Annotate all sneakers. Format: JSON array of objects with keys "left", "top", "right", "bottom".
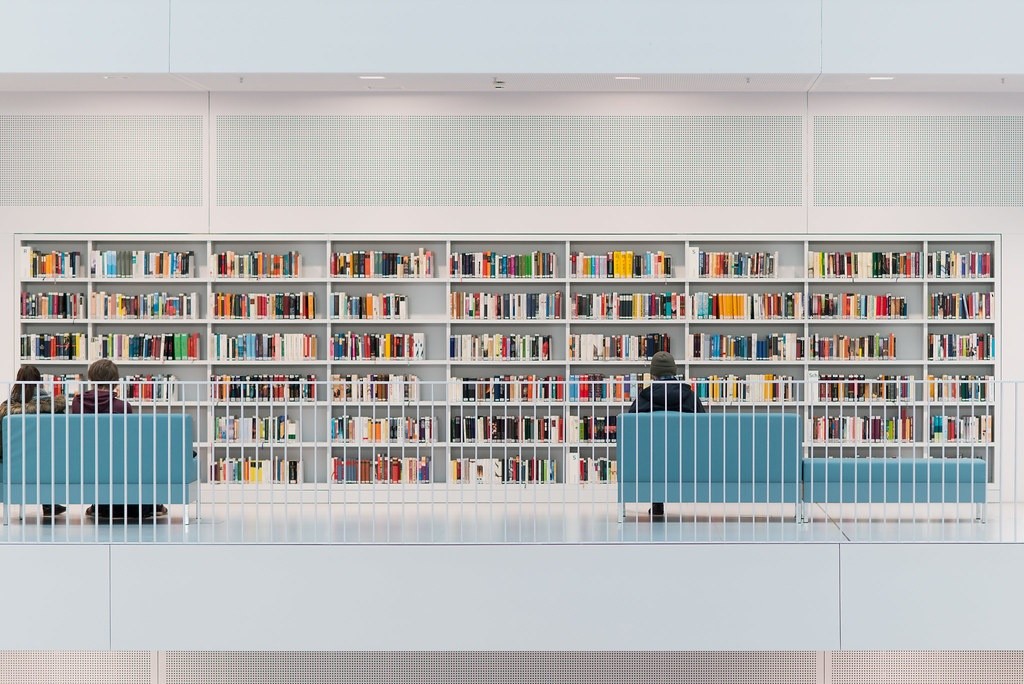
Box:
[
  {"left": 85, "top": 505, "right": 102, "bottom": 516},
  {"left": 648, "top": 503, "right": 664, "bottom": 515},
  {"left": 43, "top": 505, "right": 67, "bottom": 516},
  {"left": 154, "top": 506, "right": 168, "bottom": 515}
]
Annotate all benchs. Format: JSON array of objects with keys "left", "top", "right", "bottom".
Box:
[
  {"left": 1, "top": 413, "right": 201, "bottom": 525},
  {"left": 616, "top": 413, "right": 803, "bottom": 523},
  {"left": 802, "top": 458, "right": 988, "bottom": 524}
]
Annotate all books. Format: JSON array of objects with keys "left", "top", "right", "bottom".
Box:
[{"left": 19, "top": 248, "right": 997, "bottom": 483}]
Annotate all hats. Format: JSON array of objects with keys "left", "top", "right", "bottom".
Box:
[{"left": 650, "top": 351, "right": 677, "bottom": 376}]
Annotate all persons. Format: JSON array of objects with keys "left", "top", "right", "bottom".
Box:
[
  {"left": 0, "top": 365, "right": 67, "bottom": 517},
  {"left": 71, "top": 358, "right": 167, "bottom": 519},
  {"left": 628, "top": 350, "right": 706, "bottom": 516}
]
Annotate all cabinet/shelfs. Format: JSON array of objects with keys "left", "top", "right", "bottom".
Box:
[
  {"left": 329, "top": 233, "right": 450, "bottom": 506},
  {"left": 13, "top": 233, "right": 91, "bottom": 413},
  {"left": 806, "top": 235, "right": 927, "bottom": 457},
  {"left": 927, "top": 234, "right": 1002, "bottom": 490},
  {"left": 211, "top": 233, "right": 331, "bottom": 505},
  {"left": 566, "top": 236, "right": 686, "bottom": 504},
  {"left": 448, "top": 236, "right": 566, "bottom": 506},
  {"left": 685, "top": 236, "right": 808, "bottom": 458},
  {"left": 90, "top": 233, "right": 212, "bottom": 504}
]
